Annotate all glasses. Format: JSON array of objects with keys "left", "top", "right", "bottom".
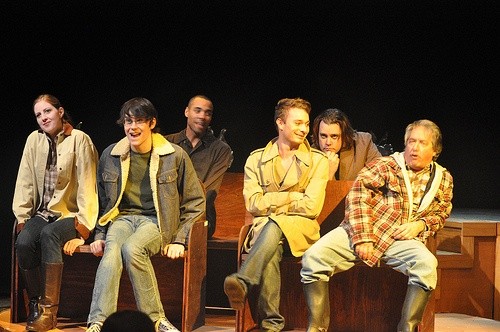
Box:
[{"left": 122, "top": 117, "right": 151, "bottom": 126}]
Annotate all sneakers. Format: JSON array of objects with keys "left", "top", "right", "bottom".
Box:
[
  {"left": 154, "top": 318, "right": 181, "bottom": 332},
  {"left": 85, "top": 321, "right": 102, "bottom": 332}
]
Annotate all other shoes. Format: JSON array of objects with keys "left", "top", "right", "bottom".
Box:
[
  {"left": 247, "top": 325, "right": 274, "bottom": 332},
  {"left": 224, "top": 275, "right": 249, "bottom": 310}
]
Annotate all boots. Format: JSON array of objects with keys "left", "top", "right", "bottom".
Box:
[
  {"left": 25, "top": 263, "right": 63, "bottom": 332},
  {"left": 18, "top": 264, "right": 42, "bottom": 324},
  {"left": 302, "top": 281, "right": 330, "bottom": 332},
  {"left": 397, "top": 284, "right": 432, "bottom": 332}
]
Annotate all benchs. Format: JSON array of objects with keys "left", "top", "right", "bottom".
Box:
[
  {"left": 235, "top": 181, "right": 435, "bottom": 332},
  {"left": 10, "top": 178, "right": 207, "bottom": 332}
]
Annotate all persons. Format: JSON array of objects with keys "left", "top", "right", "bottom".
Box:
[
  {"left": 166, "top": 95, "right": 233, "bottom": 239},
  {"left": 301, "top": 120, "right": 453, "bottom": 332},
  {"left": 311, "top": 111, "right": 383, "bottom": 181},
  {"left": 86, "top": 99, "right": 206, "bottom": 332},
  {"left": 12, "top": 94, "right": 99, "bottom": 332},
  {"left": 224, "top": 99, "right": 329, "bottom": 332},
  {"left": 102, "top": 310, "right": 156, "bottom": 332}
]
